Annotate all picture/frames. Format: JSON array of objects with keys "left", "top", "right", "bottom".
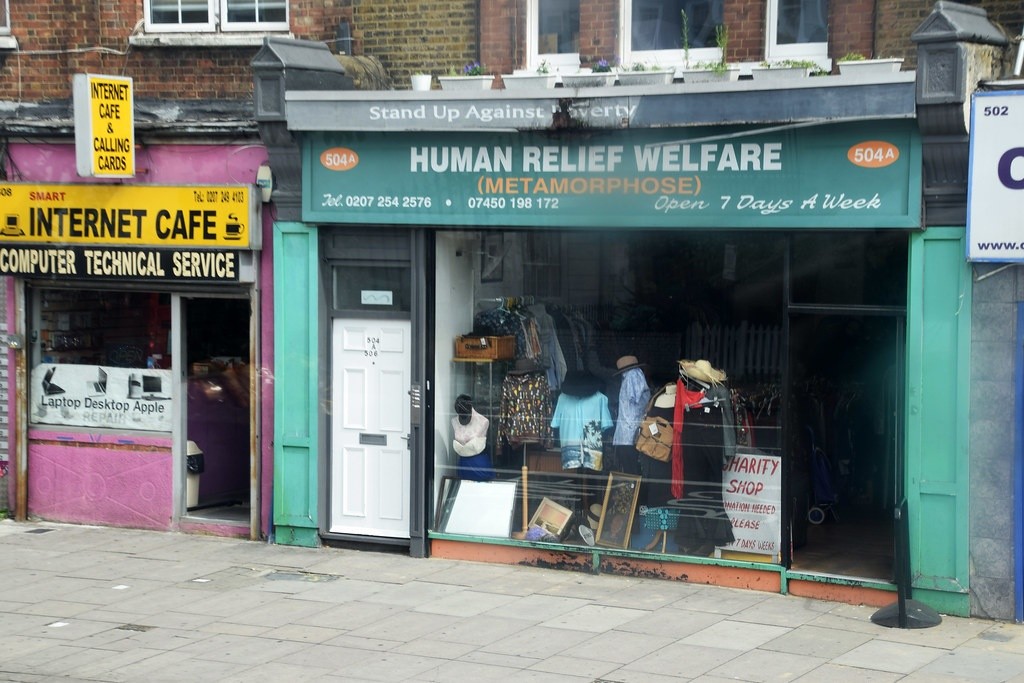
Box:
[
  {"left": 595, "top": 470, "right": 642, "bottom": 549},
  {"left": 481, "top": 232, "right": 504, "bottom": 283},
  {"left": 528, "top": 497, "right": 573, "bottom": 535}
]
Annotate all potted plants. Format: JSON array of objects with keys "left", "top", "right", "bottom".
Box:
[
  {"left": 835, "top": 50, "right": 905, "bottom": 75},
  {"left": 681, "top": 9, "right": 740, "bottom": 84},
  {"left": 500, "top": 58, "right": 560, "bottom": 90},
  {"left": 751, "top": 57, "right": 828, "bottom": 79},
  {"left": 410, "top": 71, "right": 432, "bottom": 91}
]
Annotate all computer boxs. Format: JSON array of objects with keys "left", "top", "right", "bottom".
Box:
[{"left": 128, "top": 373, "right": 142, "bottom": 399}]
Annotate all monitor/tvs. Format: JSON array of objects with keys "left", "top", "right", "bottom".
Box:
[{"left": 143, "top": 375, "right": 162, "bottom": 397}]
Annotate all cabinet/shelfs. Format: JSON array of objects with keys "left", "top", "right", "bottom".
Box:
[{"left": 40, "top": 309, "right": 108, "bottom": 356}]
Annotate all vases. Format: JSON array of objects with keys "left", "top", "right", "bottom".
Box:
[
  {"left": 438, "top": 75, "right": 496, "bottom": 91},
  {"left": 618, "top": 71, "right": 675, "bottom": 86},
  {"left": 560, "top": 74, "right": 616, "bottom": 88}
]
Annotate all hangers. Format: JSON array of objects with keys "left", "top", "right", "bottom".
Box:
[
  {"left": 492, "top": 296, "right": 583, "bottom": 320},
  {"left": 731, "top": 382, "right": 780, "bottom": 420},
  {"left": 685, "top": 389, "right": 727, "bottom": 412}
]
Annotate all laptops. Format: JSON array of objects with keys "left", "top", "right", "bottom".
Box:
[
  {"left": 86, "top": 367, "right": 108, "bottom": 395},
  {"left": 42, "top": 367, "right": 65, "bottom": 395}
]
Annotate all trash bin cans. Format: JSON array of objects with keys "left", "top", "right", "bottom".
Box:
[{"left": 187, "top": 440, "right": 203, "bottom": 508}]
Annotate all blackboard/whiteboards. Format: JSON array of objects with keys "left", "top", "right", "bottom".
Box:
[{"left": 714, "top": 453, "right": 782, "bottom": 554}]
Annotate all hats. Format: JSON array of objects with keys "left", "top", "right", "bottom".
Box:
[
  {"left": 655, "top": 385, "right": 677, "bottom": 408},
  {"left": 561, "top": 369, "right": 601, "bottom": 397},
  {"left": 588, "top": 504, "right": 601, "bottom": 530},
  {"left": 507, "top": 359, "right": 545, "bottom": 377},
  {"left": 454, "top": 394, "right": 473, "bottom": 425},
  {"left": 612, "top": 356, "right": 646, "bottom": 378},
  {"left": 680, "top": 359, "right": 727, "bottom": 382}
]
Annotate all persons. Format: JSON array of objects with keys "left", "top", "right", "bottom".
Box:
[
  {"left": 638, "top": 384, "right": 703, "bottom": 551},
  {"left": 452, "top": 394, "right": 489, "bottom": 456},
  {"left": 612, "top": 356, "right": 651, "bottom": 472},
  {"left": 551, "top": 370, "right": 613, "bottom": 472},
  {"left": 496, "top": 359, "right": 555, "bottom": 456}
]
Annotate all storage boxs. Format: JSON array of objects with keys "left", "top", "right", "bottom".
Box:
[{"left": 455, "top": 335, "right": 517, "bottom": 360}]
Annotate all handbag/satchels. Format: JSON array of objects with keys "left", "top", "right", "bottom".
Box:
[{"left": 634, "top": 416, "right": 674, "bottom": 462}]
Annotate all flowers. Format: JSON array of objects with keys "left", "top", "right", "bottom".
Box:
[
  {"left": 592, "top": 58, "right": 612, "bottom": 73},
  {"left": 445, "top": 61, "right": 486, "bottom": 76},
  {"left": 619, "top": 54, "right": 676, "bottom": 71}
]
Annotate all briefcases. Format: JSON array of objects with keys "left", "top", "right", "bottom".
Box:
[{"left": 455, "top": 335, "right": 516, "bottom": 359}]
